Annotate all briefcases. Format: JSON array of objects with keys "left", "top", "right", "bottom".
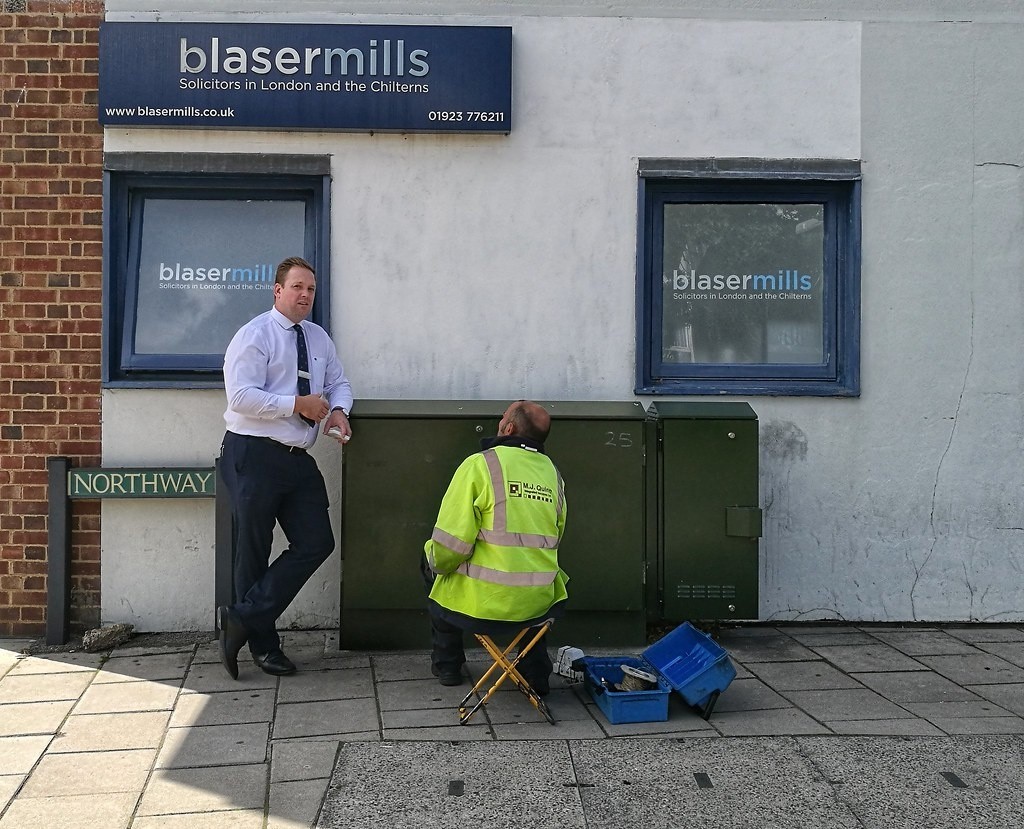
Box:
[{"left": 580, "top": 620, "right": 738, "bottom": 727}]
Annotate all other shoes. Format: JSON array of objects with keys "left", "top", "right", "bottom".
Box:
[
  {"left": 524, "top": 679, "right": 550, "bottom": 696},
  {"left": 431, "top": 664, "right": 462, "bottom": 686}
]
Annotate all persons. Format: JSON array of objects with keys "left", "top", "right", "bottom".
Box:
[
  {"left": 216, "top": 258, "right": 354, "bottom": 679},
  {"left": 420, "top": 399, "right": 571, "bottom": 698}
]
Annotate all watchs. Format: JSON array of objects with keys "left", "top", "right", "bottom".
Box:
[{"left": 330, "top": 406, "right": 350, "bottom": 419}]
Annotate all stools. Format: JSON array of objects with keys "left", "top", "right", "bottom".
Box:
[{"left": 455, "top": 618, "right": 561, "bottom": 731}]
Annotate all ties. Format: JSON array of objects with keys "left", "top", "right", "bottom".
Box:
[{"left": 292, "top": 324, "right": 315, "bottom": 428}]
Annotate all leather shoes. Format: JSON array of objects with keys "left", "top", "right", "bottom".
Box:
[
  {"left": 252, "top": 649, "right": 296, "bottom": 675},
  {"left": 217, "top": 605, "right": 247, "bottom": 679}
]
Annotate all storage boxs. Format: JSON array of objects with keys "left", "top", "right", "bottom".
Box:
[{"left": 582, "top": 618, "right": 741, "bottom": 725}]
